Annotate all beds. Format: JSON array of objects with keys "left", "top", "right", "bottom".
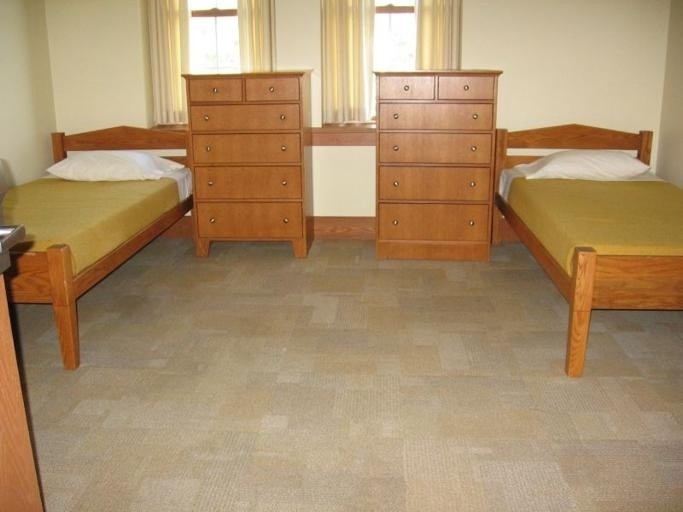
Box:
[
  {"left": 0, "top": 122, "right": 193, "bottom": 373},
  {"left": 488, "top": 123, "right": 682, "bottom": 384}
]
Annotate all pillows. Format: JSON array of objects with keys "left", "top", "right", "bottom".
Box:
[
  {"left": 45, "top": 149, "right": 185, "bottom": 184},
  {"left": 512, "top": 143, "right": 655, "bottom": 184}
]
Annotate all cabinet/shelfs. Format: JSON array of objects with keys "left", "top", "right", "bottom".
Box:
[
  {"left": 175, "top": 64, "right": 318, "bottom": 259},
  {"left": 368, "top": 66, "right": 505, "bottom": 266}
]
